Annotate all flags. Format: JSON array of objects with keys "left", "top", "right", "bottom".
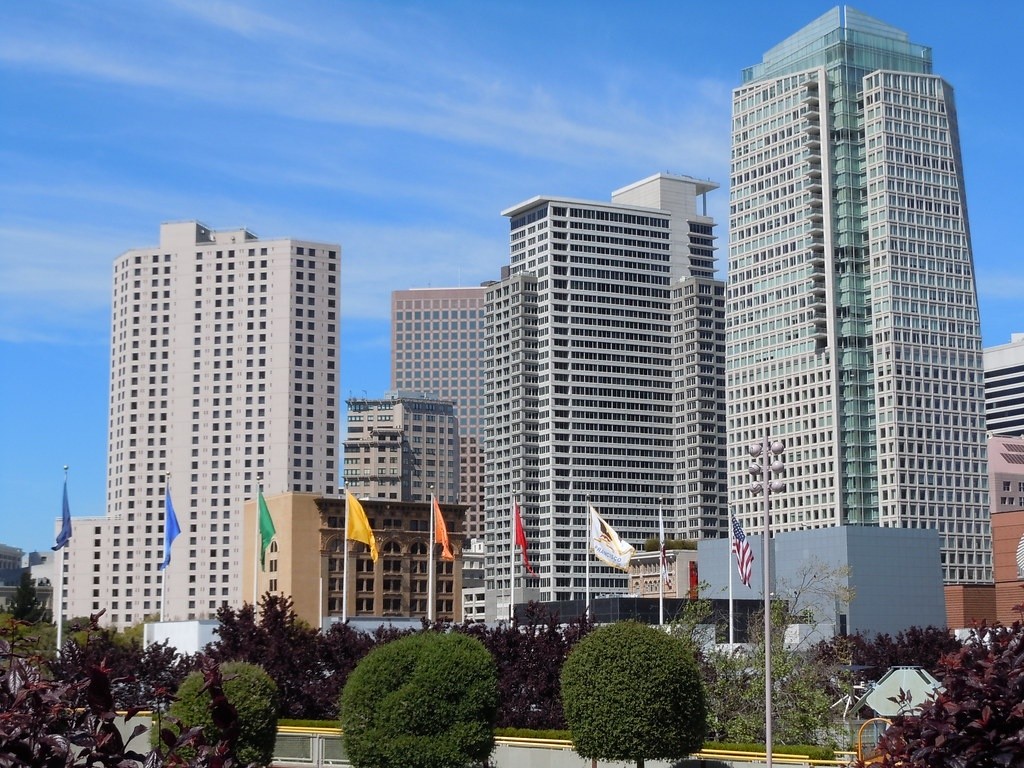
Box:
[
  {"left": 515, "top": 504, "right": 528, "bottom": 565},
  {"left": 345, "top": 491, "right": 378, "bottom": 564},
  {"left": 660, "top": 511, "right": 672, "bottom": 589},
  {"left": 589, "top": 506, "right": 635, "bottom": 572},
  {"left": 258, "top": 492, "right": 275, "bottom": 572},
  {"left": 161, "top": 486, "right": 181, "bottom": 570},
  {"left": 731, "top": 510, "right": 754, "bottom": 589},
  {"left": 51, "top": 483, "right": 72, "bottom": 551},
  {"left": 434, "top": 497, "right": 455, "bottom": 560}
]
